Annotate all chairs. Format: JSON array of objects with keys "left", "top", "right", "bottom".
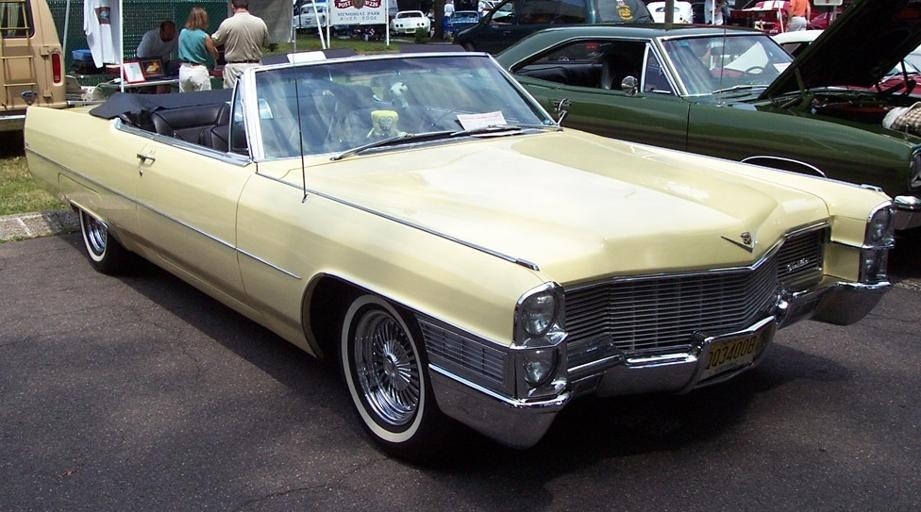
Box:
[
  {"left": 519, "top": 44, "right": 736, "bottom": 99},
  {"left": 144, "top": 82, "right": 414, "bottom": 159}
]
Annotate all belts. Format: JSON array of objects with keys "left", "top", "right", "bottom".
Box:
[
  {"left": 227, "top": 59, "right": 259, "bottom": 63},
  {"left": 179, "top": 59, "right": 203, "bottom": 65}
]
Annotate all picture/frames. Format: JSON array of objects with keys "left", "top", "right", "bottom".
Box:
[{"left": 122, "top": 57, "right": 168, "bottom": 83}]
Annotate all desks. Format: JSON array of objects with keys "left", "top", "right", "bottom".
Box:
[{"left": 101, "top": 76, "right": 185, "bottom": 102}]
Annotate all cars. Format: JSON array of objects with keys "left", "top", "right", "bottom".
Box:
[
  {"left": 292, "top": 0, "right": 433, "bottom": 40},
  {"left": 23, "top": 47, "right": 900, "bottom": 455}
]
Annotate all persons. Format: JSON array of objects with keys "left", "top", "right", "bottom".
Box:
[
  {"left": 137, "top": 0, "right": 272, "bottom": 93},
  {"left": 443, "top": 0, "right": 455, "bottom": 33},
  {"left": 425, "top": 9, "right": 434, "bottom": 19},
  {"left": 786, "top": 0, "right": 811, "bottom": 32},
  {"left": 704, "top": 1, "right": 725, "bottom": 26},
  {"left": 478, "top": 0, "right": 486, "bottom": 18}
]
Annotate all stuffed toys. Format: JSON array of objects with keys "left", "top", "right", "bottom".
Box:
[{"left": 364, "top": 81, "right": 408, "bottom": 139}]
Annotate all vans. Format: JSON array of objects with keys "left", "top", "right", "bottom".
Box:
[{"left": 0, "top": 0, "right": 69, "bottom": 134}]
